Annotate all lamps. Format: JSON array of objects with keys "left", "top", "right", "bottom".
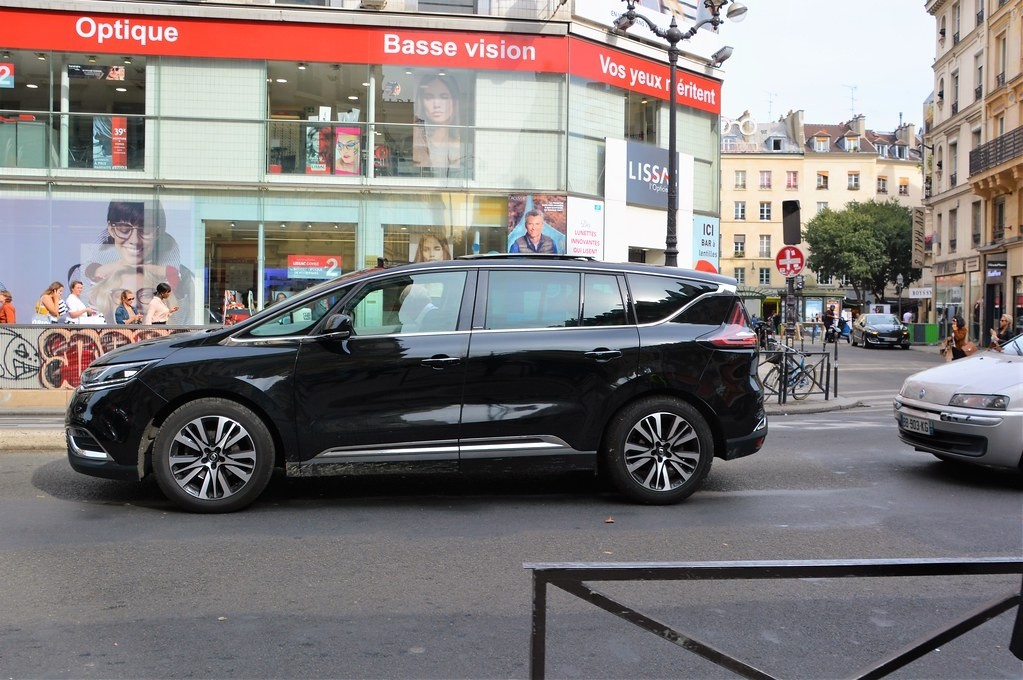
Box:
[
  {"left": 332, "top": 65, "right": 340, "bottom": 72},
  {"left": 122, "top": 57, "right": 132, "bottom": 64},
  {"left": 707, "top": 46, "right": 734, "bottom": 68},
  {"left": 298, "top": 63, "right": 305, "bottom": 70},
  {"left": 3, "top": 51, "right": 10, "bottom": 59},
  {"left": 38, "top": 53, "right": 46, "bottom": 61},
  {"left": 360, "top": 0, "right": 388, "bottom": 10},
  {"left": 641, "top": 98, "right": 647, "bottom": 104},
  {"left": 89, "top": 56, "right": 96, "bottom": 63}
]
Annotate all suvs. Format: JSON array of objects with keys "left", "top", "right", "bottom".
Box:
[{"left": 65, "top": 254, "right": 768, "bottom": 514}]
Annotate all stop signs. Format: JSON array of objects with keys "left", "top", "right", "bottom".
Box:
[{"left": 775, "top": 246, "right": 805, "bottom": 277}]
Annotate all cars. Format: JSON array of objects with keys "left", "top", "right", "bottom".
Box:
[
  {"left": 849, "top": 313, "right": 911, "bottom": 350},
  {"left": 893, "top": 333, "right": 1023, "bottom": 471}
]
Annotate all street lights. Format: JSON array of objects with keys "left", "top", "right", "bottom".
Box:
[{"left": 613, "top": 0, "right": 748, "bottom": 267}]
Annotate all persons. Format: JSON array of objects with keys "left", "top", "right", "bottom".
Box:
[
  {"left": 306, "top": 126, "right": 331, "bottom": 168},
  {"left": 903, "top": 309, "right": 913, "bottom": 323},
  {"left": 0, "top": 290, "right": 16, "bottom": 324},
  {"left": 336, "top": 131, "right": 361, "bottom": 172},
  {"left": 390, "top": 84, "right": 400, "bottom": 96},
  {"left": 509, "top": 209, "right": 558, "bottom": 253},
  {"left": 414, "top": 73, "right": 460, "bottom": 169},
  {"left": 64, "top": 281, "right": 95, "bottom": 324},
  {"left": 91, "top": 265, "right": 179, "bottom": 325},
  {"left": 989, "top": 314, "right": 1015, "bottom": 349},
  {"left": 412, "top": 230, "right": 451, "bottom": 262},
  {"left": 399, "top": 284, "right": 441, "bottom": 332},
  {"left": 145, "top": 282, "right": 179, "bottom": 326},
  {"left": 115, "top": 290, "right": 144, "bottom": 326},
  {"left": 990, "top": 328, "right": 999, "bottom": 346},
  {"left": 973, "top": 301, "right": 980, "bottom": 341},
  {"left": 68, "top": 199, "right": 195, "bottom": 325},
  {"left": 276, "top": 293, "right": 287, "bottom": 303},
  {"left": 107, "top": 66, "right": 120, "bottom": 81},
  {"left": 31, "top": 282, "right": 68, "bottom": 325},
  {"left": 822, "top": 305, "right": 841, "bottom": 342},
  {"left": 940, "top": 315, "right": 968, "bottom": 361}
]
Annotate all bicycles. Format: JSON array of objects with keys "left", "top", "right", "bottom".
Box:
[{"left": 763, "top": 341, "right": 817, "bottom": 402}]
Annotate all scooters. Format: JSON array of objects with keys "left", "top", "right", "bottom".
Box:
[
  {"left": 828, "top": 317, "right": 850, "bottom": 343},
  {"left": 753, "top": 320, "right": 774, "bottom": 349}
]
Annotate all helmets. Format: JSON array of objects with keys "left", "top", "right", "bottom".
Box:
[{"left": 830, "top": 305, "right": 835, "bottom": 312}]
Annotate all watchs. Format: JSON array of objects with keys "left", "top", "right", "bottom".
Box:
[{"left": 854, "top": 313, "right": 859, "bottom": 320}]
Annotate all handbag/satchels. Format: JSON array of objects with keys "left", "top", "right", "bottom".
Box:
[
  {"left": 961, "top": 333, "right": 977, "bottom": 357},
  {"left": 33, "top": 314, "right": 51, "bottom": 325},
  {"left": 81, "top": 310, "right": 106, "bottom": 325}
]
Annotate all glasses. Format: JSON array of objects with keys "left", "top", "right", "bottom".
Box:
[
  {"left": 336, "top": 139, "right": 358, "bottom": 153},
  {"left": 111, "top": 287, "right": 156, "bottom": 305},
  {"left": 999, "top": 319, "right": 1007, "bottom": 323},
  {"left": 952, "top": 319, "right": 957, "bottom": 323},
  {"left": 125, "top": 297, "right": 135, "bottom": 302},
  {"left": 108, "top": 220, "right": 162, "bottom": 240}
]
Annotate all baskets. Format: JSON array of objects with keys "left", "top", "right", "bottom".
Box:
[{"left": 766, "top": 346, "right": 784, "bottom": 364}]
